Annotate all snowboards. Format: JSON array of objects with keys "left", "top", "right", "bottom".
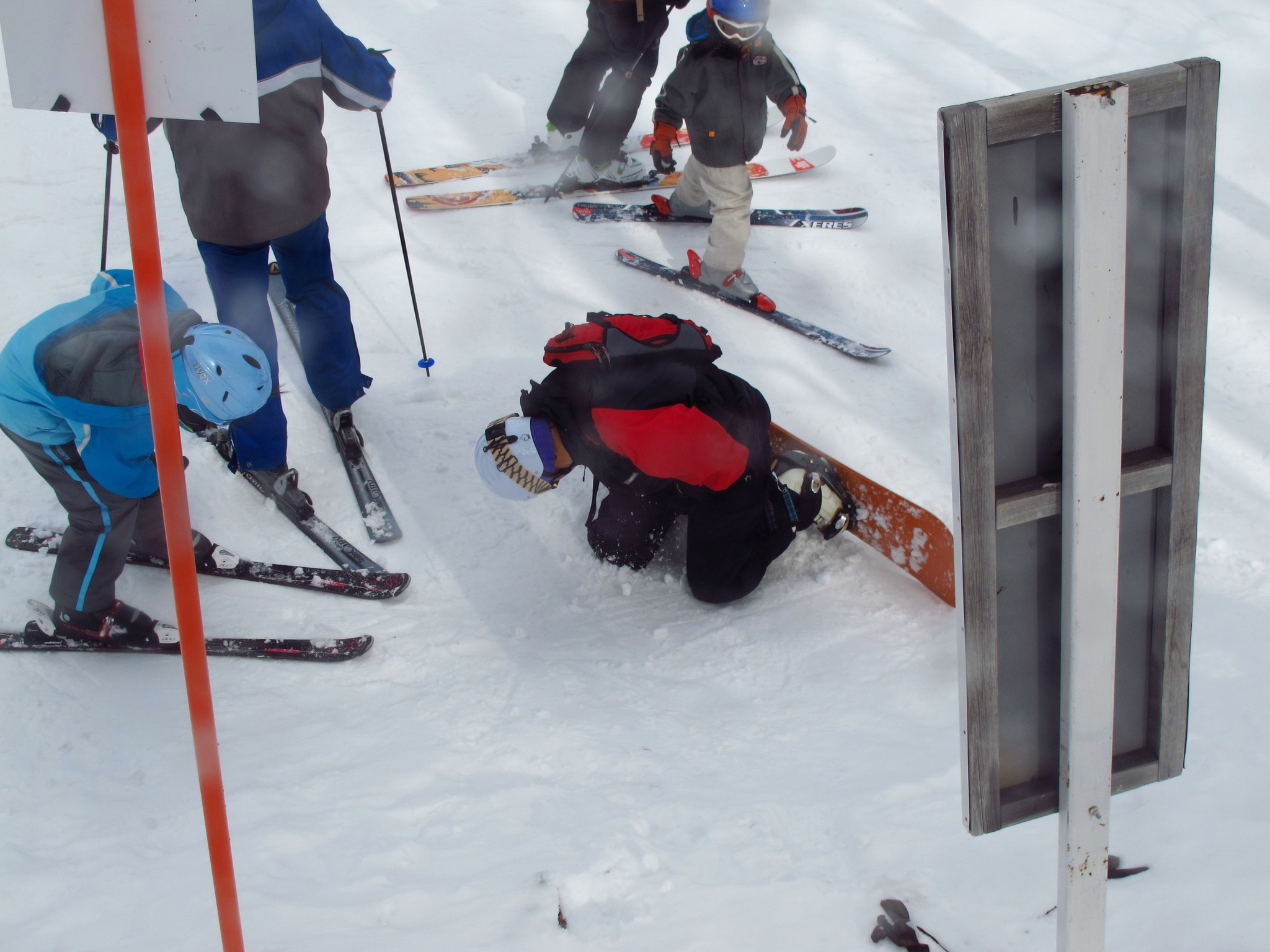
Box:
[{"left": 770, "top": 420, "right": 956, "bottom": 608}]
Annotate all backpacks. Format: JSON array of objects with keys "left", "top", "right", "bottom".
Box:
[{"left": 543, "top": 312, "right": 723, "bottom": 449}]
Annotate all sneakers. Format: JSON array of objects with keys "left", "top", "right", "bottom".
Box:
[
  {"left": 574, "top": 146, "right": 646, "bottom": 185},
  {"left": 544, "top": 120, "right": 587, "bottom": 152},
  {"left": 128, "top": 523, "right": 212, "bottom": 565},
  {"left": 774, "top": 450, "right": 849, "bottom": 540},
  {"left": 668, "top": 189, "right": 717, "bottom": 219},
  {"left": 697, "top": 261, "right": 759, "bottom": 302},
  {"left": 54, "top": 599, "right": 154, "bottom": 644}
]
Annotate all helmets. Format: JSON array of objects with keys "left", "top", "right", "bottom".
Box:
[
  {"left": 171, "top": 322, "right": 272, "bottom": 424},
  {"left": 706, "top": 0, "right": 770, "bottom": 30},
  {"left": 475, "top": 417, "right": 555, "bottom": 502}
]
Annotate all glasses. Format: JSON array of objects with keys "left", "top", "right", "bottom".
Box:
[{"left": 713, "top": 14, "right": 764, "bottom": 41}]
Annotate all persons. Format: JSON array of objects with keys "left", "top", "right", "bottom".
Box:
[
  {"left": 0, "top": 267, "right": 272, "bottom": 642},
  {"left": 475, "top": 360, "right": 851, "bottom": 603},
  {"left": 529, "top": 0, "right": 691, "bottom": 186},
  {"left": 650, "top": 0, "right": 808, "bottom": 301},
  {"left": 90, "top": 0, "right": 395, "bottom": 473}
]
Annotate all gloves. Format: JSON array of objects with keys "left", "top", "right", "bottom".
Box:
[
  {"left": 649, "top": 122, "right": 680, "bottom": 175},
  {"left": 780, "top": 94, "right": 816, "bottom": 152}
]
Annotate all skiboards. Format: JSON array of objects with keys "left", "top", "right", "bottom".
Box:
[
  {"left": 0, "top": 526, "right": 411, "bottom": 663},
  {"left": 192, "top": 262, "right": 402, "bottom": 574},
  {"left": 384, "top": 104, "right": 836, "bottom": 210},
  {"left": 573, "top": 201, "right": 891, "bottom": 359}
]
